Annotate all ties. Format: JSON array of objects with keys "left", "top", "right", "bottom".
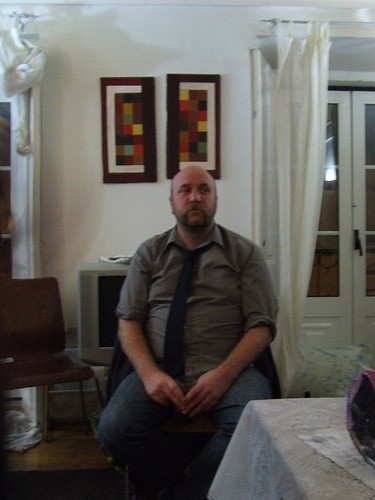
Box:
[{"left": 163, "top": 241, "right": 216, "bottom": 383}]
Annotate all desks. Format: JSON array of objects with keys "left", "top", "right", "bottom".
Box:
[{"left": 206, "top": 396, "right": 375, "bottom": 500}]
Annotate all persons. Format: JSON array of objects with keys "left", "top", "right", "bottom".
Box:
[{"left": 99, "top": 166, "right": 277, "bottom": 500}]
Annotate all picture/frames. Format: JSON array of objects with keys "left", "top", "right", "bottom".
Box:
[
  {"left": 99, "top": 76, "right": 157, "bottom": 183},
  {"left": 166, "top": 73, "right": 220, "bottom": 181}
]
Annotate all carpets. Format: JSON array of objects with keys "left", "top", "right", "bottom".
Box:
[{"left": 0, "top": 468, "right": 134, "bottom": 500}]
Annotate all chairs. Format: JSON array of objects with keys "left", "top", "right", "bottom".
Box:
[
  {"left": 106, "top": 341, "right": 283, "bottom": 500},
  {"left": 0, "top": 276, "right": 104, "bottom": 424}
]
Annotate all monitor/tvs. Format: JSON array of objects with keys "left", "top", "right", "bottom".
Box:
[{"left": 78, "top": 262, "right": 128, "bottom": 367}]
